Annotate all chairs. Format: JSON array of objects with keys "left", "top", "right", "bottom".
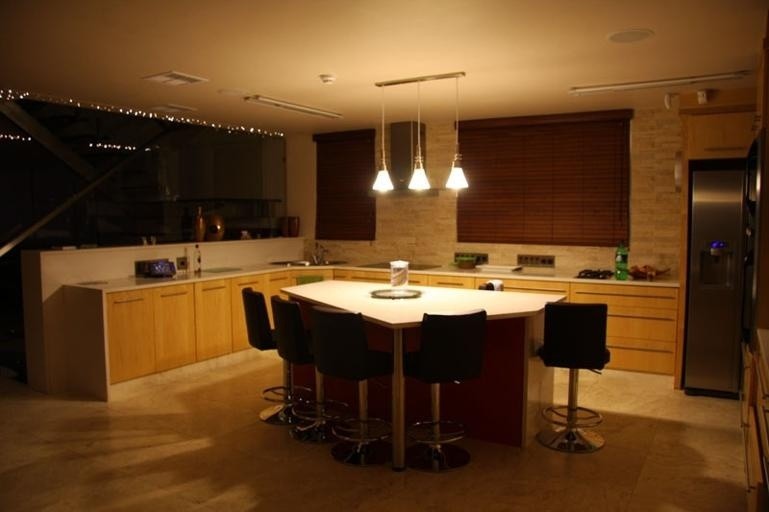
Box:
[
  {"left": 240, "top": 286, "right": 306, "bottom": 427},
  {"left": 310, "top": 303, "right": 394, "bottom": 466},
  {"left": 405, "top": 309, "right": 488, "bottom": 474},
  {"left": 536, "top": 302, "right": 609, "bottom": 453},
  {"left": 270, "top": 295, "right": 339, "bottom": 446}
]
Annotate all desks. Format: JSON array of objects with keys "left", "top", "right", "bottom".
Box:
[{"left": 281, "top": 279, "right": 568, "bottom": 448}]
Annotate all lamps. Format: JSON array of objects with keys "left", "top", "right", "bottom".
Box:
[
  {"left": 445, "top": 77, "right": 469, "bottom": 190},
  {"left": 143, "top": 69, "right": 208, "bottom": 89},
  {"left": 570, "top": 72, "right": 747, "bottom": 97},
  {"left": 372, "top": 85, "right": 395, "bottom": 192},
  {"left": 408, "top": 80, "right": 432, "bottom": 192},
  {"left": 246, "top": 96, "right": 344, "bottom": 120}
]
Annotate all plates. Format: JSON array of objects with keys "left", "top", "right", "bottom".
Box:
[
  {"left": 371, "top": 289, "right": 421, "bottom": 299},
  {"left": 629, "top": 265, "right": 670, "bottom": 281}
]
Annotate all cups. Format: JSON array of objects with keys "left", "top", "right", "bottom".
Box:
[{"left": 389, "top": 261, "right": 409, "bottom": 296}]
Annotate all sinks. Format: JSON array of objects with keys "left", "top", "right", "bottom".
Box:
[{"left": 272, "top": 261, "right": 347, "bottom": 266}]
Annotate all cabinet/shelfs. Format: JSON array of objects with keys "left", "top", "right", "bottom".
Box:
[
  {"left": 740, "top": 328, "right": 769, "bottom": 512},
  {"left": 75, "top": 260, "right": 333, "bottom": 402},
  {"left": 336, "top": 262, "right": 680, "bottom": 374}
]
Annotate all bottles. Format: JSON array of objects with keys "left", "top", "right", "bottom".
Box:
[
  {"left": 614, "top": 239, "right": 629, "bottom": 281},
  {"left": 193, "top": 244, "right": 202, "bottom": 273},
  {"left": 195, "top": 206, "right": 207, "bottom": 242}
]
